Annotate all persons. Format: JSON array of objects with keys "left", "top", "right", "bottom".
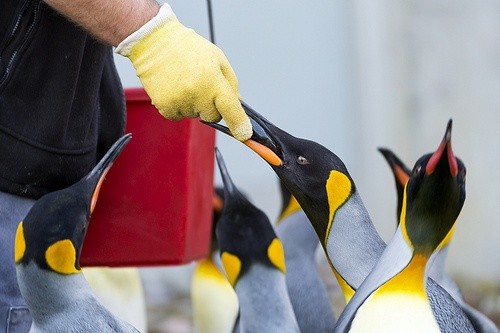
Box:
[{"left": 1, "top": 0, "right": 254, "bottom": 333}]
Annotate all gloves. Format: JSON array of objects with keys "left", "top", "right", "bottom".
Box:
[{"left": 112, "top": 4, "right": 254, "bottom": 142}]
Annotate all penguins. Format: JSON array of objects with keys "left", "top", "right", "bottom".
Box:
[
  {"left": 143, "top": 147, "right": 347, "bottom": 333},
  {"left": 199, "top": 98, "right": 477, "bottom": 333},
  {"left": 377, "top": 148, "right": 500, "bottom": 333},
  {"left": 14, "top": 132, "right": 141, "bottom": 333},
  {"left": 330, "top": 118, "right": 477, "bottom": 333}
]
